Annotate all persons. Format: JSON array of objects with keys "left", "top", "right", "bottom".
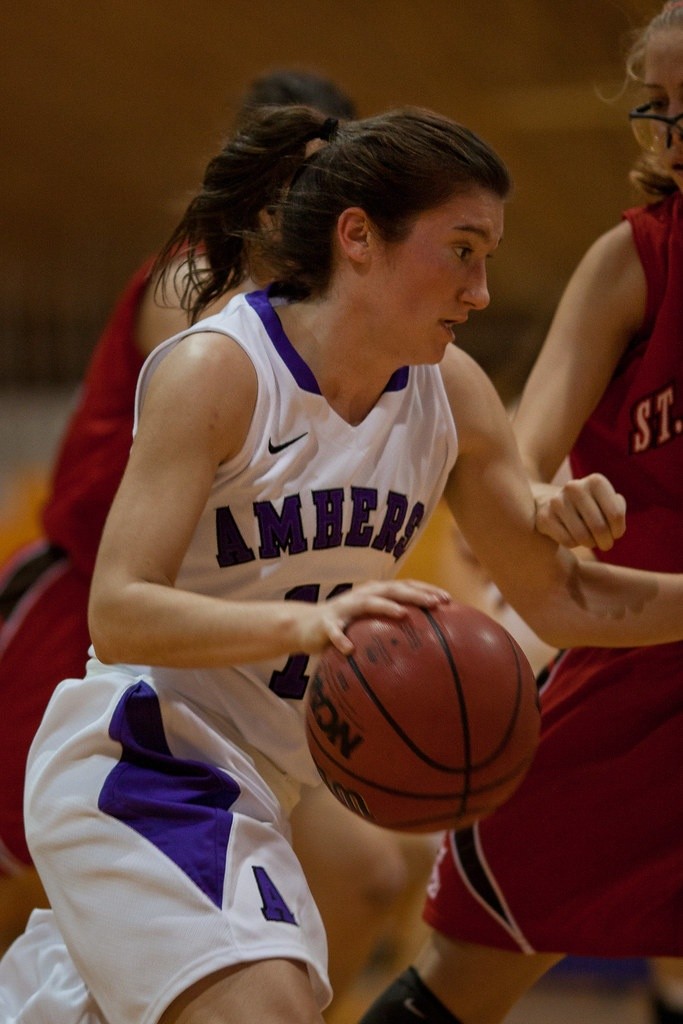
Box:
[
  {"left": 0, "top": 72, "right": 406, "bottom": 1024},
  {"left": 358, "top": 1, "right": 683, "bottom": 1022},
  {"left": 1, "top": 101, "right": 683, "bottom": 1024}
]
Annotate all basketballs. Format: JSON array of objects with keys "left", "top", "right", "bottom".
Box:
[{"left": 301, "top": 596, "right": 544, "bottom": 838}]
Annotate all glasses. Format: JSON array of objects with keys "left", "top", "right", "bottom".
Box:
[{"left": 628, "top": 101, "right": 683, "bottom": 154}]
